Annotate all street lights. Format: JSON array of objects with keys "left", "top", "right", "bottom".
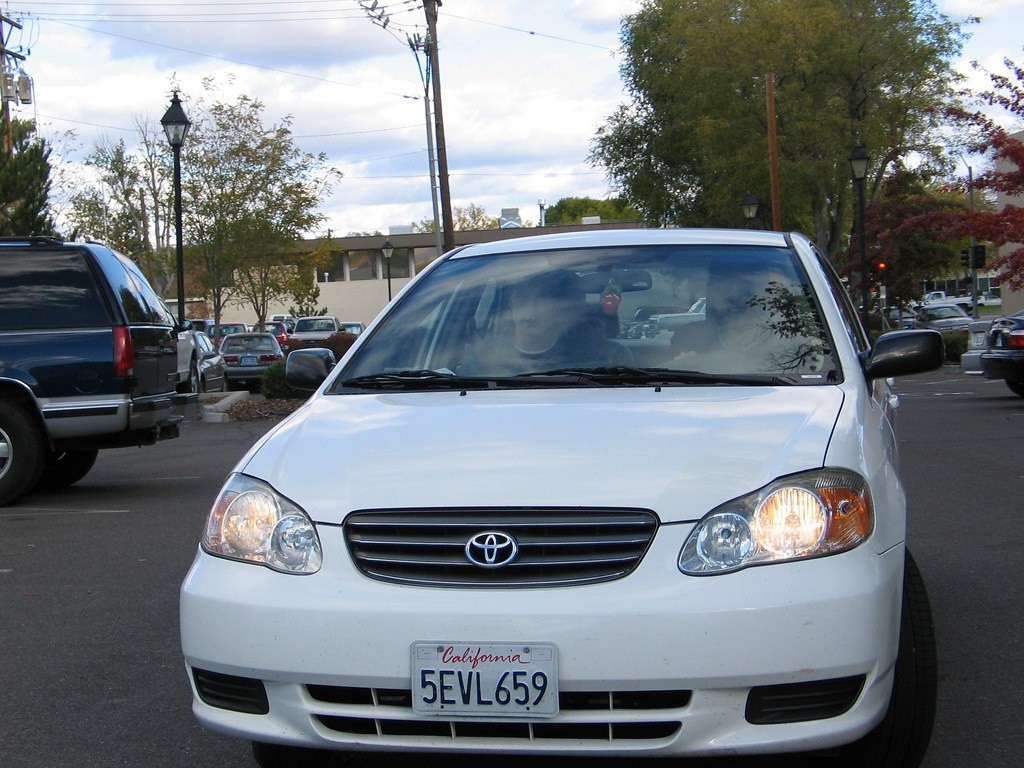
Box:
[
  {"left": 160, "top": 91, "right": 193, "bottom": 326},
  {"left": 846, "top": 143, "right": 872, "bottom": 340},
  {"left": 380, "top": 236, "right": 396, "bottom": 305},
  {"left": 738, "top": 194, "right": 759, "bottom": 228}
]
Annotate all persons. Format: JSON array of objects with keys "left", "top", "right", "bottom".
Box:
[
  {"left": 452, "top": 266, "right": 610, "bottom": 379},
  {"left": 668, "top": 261, "right": 810, "bottom": 376}
]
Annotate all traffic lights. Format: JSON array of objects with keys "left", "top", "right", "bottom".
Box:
[{"left": 960, "top": 249, "right": 969, "bottom": 266}]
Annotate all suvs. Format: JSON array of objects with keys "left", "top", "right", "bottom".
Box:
[{"left": 0, "top": 231, "right": 200, "bottom": 509}]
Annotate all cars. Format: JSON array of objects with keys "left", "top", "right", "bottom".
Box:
[
  {"left": 176, "top": 221, "right": 952, "bottom": 766},
  {"left": 911, "top": 302, "right": 975, "bottom": 342},
  {"left": 960, "top": 319, "right": 996, "bottom": 379},
  {"left": 978, "top": 310, "right": 1024, "bottom": 399},
  {"left": 178, "top": 314, "right": 368, "bottom": 394},
  {"left": 859, "top": 289, "right": 1002, "bottom": 330}
]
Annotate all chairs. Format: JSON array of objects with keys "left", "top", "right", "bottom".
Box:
[
  {"left": 670, "top": 265, "right": 787, "bottom": 359},
  {"left": 493, "top": 270, "right": 635, "bottom": 371}
]
